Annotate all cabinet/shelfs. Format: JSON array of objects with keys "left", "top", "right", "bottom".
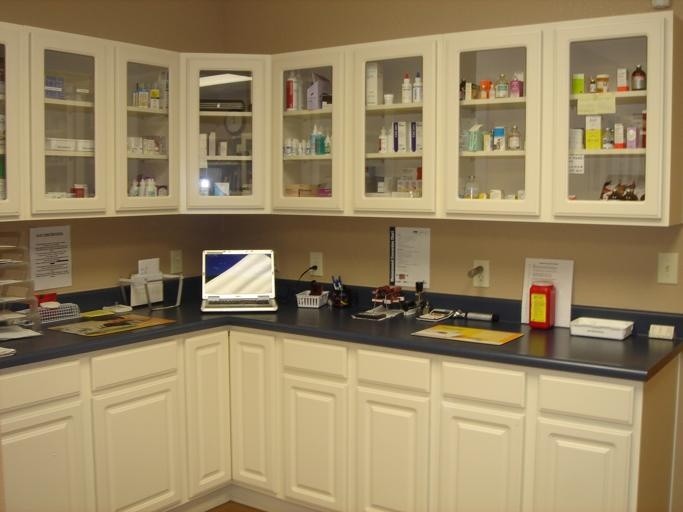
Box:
[
  {"left": 1, "top": 332, "right": 186, "bottom": 512},
  {"left": 267, "top": 34, "right": 444, "bottom": 220},
  {"left": 230, "top": 318, "right": 274, "bottom": 512},
  {"left": 0, "top": 20, "right": 29, "bottom": 223},
  {"left": 181, "top": 50, "right": 272, "bottom": 216},
  {"left": 431, "top": 344, "right": 676, "bottom": 512},
  {"left": 275, "top": 330, "right": 430, "bottom": 512},
  {"left": 27, "top": 24, "right": 185, "bottom": 222},
  {"left": 182, "top": 318, "right": 230, "bottom": 512},
  {"left": 436, "top": 10, "right": 683, "bottom": 227}
]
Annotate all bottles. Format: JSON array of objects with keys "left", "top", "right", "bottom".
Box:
[
  {"left": 462, "top": 174, "right": 478, "bottom": 199},
  {"left": 508, "top": 122, "right": 522, "bottom": 150},
  {"left": 282, "top": 123, "right": 330, "bottom": 158},
  {"left": 603, "top": 125, "right": 616, "bottom": 149},
  {"left": 286, "top": 69, "right": 303, "bottom": 112},
  {"left": 128, "top": 177, "right": 157, "bottom": 198},
  {"left": 479, "top": 74, "right": 521, "bottom": 99},
  {"left": 629, "top": 64, "right": 646, "bottom": 90},
  {"left": 401, "top": 71, "right": 421, "bottom": 103},
  {"left": 377, "top": 125, "right": 392, "bottom": 154},
  {"left": 588, "top": 77, "right": 608, "bottom": 94}
]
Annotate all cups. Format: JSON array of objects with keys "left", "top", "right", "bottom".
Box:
[{"left": 383, "top": 94, "right": 394, "bottom": 105}]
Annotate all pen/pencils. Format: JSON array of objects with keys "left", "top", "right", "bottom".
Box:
[{"left": 331, "top": 275, "right": 344, "bottom": 291}]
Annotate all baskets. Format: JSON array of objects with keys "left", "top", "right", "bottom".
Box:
[{"left": 296, "top": 290, "right": 330, "bottom": 308}]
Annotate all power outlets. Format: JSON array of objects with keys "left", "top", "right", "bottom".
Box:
[
  {"left": 473, "top": 260, "right": 490, "bottom": 287},
  {"left": 170, "top": 250, "right": 183, "bottom": 273},
  {"left": 309, "top": 252, "right": 323, "bottom": 277}
]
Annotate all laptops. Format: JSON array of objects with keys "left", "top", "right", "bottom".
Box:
[{"left": 200, "top": 249, "right": 279, "bottom": 312}]
